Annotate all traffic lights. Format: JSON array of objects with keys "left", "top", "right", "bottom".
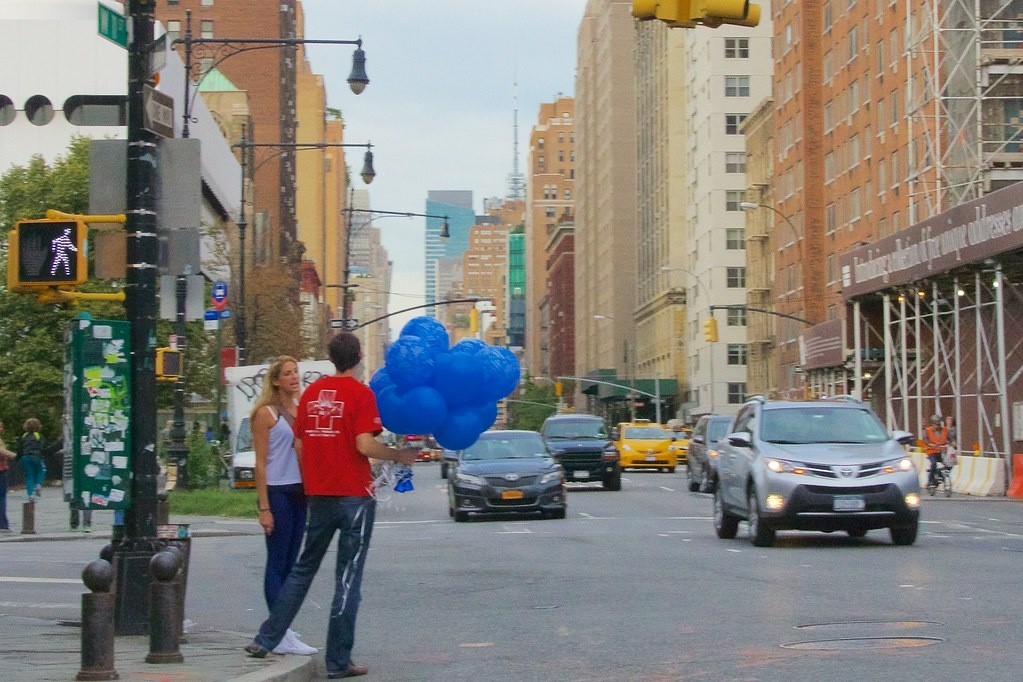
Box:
[
  {"left": 156, "top": 348, "right": 182, "bottom": 382},
  {"left": 631, "top": 0, "right": 761, "bottom": 28},
  {"left": 8, "top": 218, "right": 87, "bottom": 291},
  {"left": 703, "top": 319, "right": 718, "bottom": 342}
]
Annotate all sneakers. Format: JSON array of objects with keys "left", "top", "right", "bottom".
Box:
[{"left": 272, "top": 627, "right": 319, "bottom": 655}]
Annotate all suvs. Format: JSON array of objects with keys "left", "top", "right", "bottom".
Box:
[
  {"left": 714, "top": 395, "right": 921, "bottom": 546},
  {"left": 541, "top": 410, "right": 621, "bottom": 490}
]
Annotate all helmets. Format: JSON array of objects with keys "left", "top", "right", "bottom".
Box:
[{"left": 930, "top": 415, "right": 940, "bottom": 424}]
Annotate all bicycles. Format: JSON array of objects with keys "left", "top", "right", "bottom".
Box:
[{"left": 925, "top": 443, "right": 952, "bottom": 497}]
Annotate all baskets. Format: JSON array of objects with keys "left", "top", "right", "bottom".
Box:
[{"left": 941, "top": 449, "right": 960, "bottom": 467}]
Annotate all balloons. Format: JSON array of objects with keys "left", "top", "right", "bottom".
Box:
[{"left": 368, "top": 316, "right": 520, "bottom": 452}]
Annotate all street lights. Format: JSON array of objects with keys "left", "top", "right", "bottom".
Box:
[
  {"left": 662, "top": 267, "right": 713, "bottom": 413},
  {"left": 739, "top": 202, "right": 802, "bottom": 264},
  {"left": 594, "top": 315, "right": 634, "bottom": 423},
  {"left": 343, "top": 208, "right": 451, "bottom": 333},
  {"left": 230, "top": 122, "right": 376, "bottom": 367},
  {"left": 166, "top": 36, "right": 369, "bottom": 490}
]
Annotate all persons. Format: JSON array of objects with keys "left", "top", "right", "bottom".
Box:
[
  {"left": 0, "top": 421, "right": 17, "bottom": 532},
  {"left": 69, "top": 510, "right": 93, "bottom": 532},
  {"left": 244, "top": 332, "right": 417, "bottom": 679},
  {"left": 249, "top": 355, "right": 318, "bottom": 655},
  {"left": 922, "top": 414, "right": 957, "bottom": 488},
  {"left": 192, "top": 422, "right": 231, "bottom": 443},
  {"left": 15, "top": 417, "right": 47, "bottom": 502}
]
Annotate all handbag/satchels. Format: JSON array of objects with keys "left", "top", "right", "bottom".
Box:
[
  {"left": 945, "top": 447, "right": 956, "bottom": 462},
  {"left": 947, "top": 446, "right": 958, "bottom": 466}
]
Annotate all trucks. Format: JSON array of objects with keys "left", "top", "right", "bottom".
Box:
[{"left": 224, "top": 360, "right": 336, "bottom": 490}]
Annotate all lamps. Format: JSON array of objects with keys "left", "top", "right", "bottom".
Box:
[
  {"left": 991, "top": 276, "right": 998, "bottom": 288},
  {"left": 740, "top": 201, "right": 800, "bottom": 243},
  {"left": 958, "top": 285, "right": 966, "bottom": 297},
  {"left": 929, "top": 295, "right": 948, "bottom": 305}
]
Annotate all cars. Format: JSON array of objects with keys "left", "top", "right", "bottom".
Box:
[
  {"left": 666, "top": 428, "right": 693, "bottom": 464},
  {"left": 611, "top": 422, "right": 677, "bottom": 473},
  {"left": 440, "top": 448, "right": 458, "bottom": 479},
  {"left": 686, "top": 415, "right": 734, "bottom": 493},
  {"left": 446, "top": 429, "right": 566, "bottom": 522}
]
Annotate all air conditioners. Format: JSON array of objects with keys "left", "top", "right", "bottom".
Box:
[{"left": 280, "top": 4, "right": 289, "bottom": 13}]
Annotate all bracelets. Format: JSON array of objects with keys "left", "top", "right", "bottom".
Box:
[{"left": 260, "top": 509, "right": 270, "bottom": 510}]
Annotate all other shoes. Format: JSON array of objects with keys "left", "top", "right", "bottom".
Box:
[
  {"left": 245, "top": 643, "right": 269, "bottom": 658},
  {"left": 929, "top": 480, "right": 937, "bottom": 488},
  {"left": 36, "top": 490, "right": 41, "bottom": 498},
  {"left": 0, "top": 527, "right": 14, "bottom": 533},
  {"left": 70, "top": 511, "right": 91, "bottom": 532},
  {"left": 30, "top": 491, "right": 41, "bottom": 501},
  {"left": 29, "top": 495, "right": 35, "bottom": 501},
  {"left": 327, "top": 661, "right": 367, "bottom": 679}
]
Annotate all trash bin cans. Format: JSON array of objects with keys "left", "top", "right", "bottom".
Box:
[{"left": 112, "top": 523, "right": 191, "bottom": 636}]
